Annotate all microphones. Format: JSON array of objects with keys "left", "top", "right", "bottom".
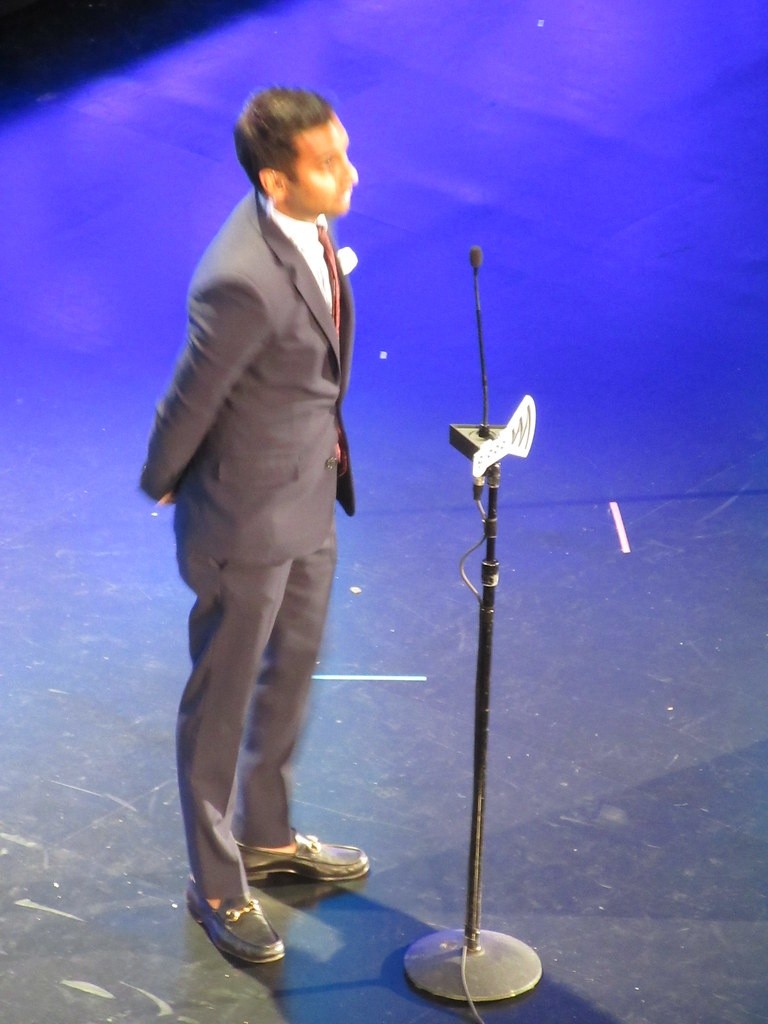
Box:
[{"left": 468, "top": 244, "right": 489, "bottom": 424}]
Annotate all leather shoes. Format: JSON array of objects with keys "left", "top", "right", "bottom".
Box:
[
  {"left": 186, "top": 873, "right": 285, "bottom": 963},
  {"left": 236, "top": 830, "right": 370, "bottom": 880}
]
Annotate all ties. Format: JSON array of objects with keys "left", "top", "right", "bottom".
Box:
[{"left": 313, "top": 224, "right": 346, "bottom": 463}]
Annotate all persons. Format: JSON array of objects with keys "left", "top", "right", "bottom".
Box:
[{"left": 137, "top": 88, "right": 368, "bottom": 963}]
